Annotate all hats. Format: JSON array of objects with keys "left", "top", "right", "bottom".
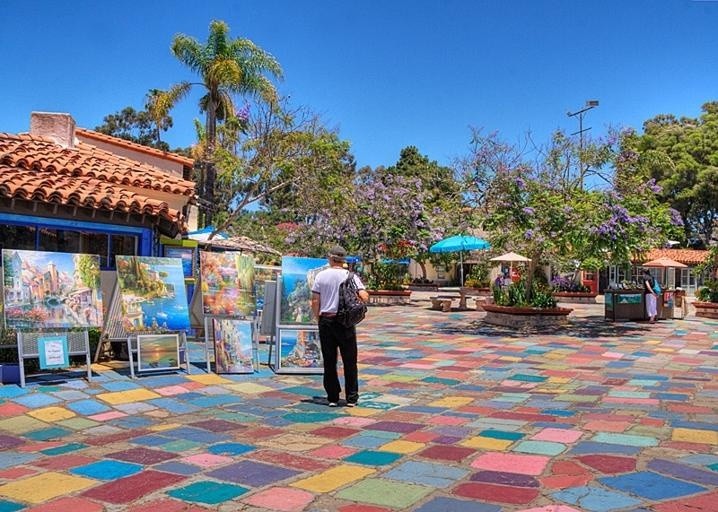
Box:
[{"left": 326, "top": 245, "right": 346, "bottom": 262}]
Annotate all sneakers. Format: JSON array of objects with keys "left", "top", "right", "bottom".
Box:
[
  {"left": 347, "top": 402, "right": 356, "bottom": 406},
  {"left": 328, "top": 402, "right": 337, "bottom": 406}
]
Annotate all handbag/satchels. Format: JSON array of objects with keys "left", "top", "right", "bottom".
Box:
[{"left": 653, "top": 278, "right": 661, "bottom": 295}]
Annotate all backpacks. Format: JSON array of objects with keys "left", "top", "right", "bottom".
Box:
[{"left": 338, "top": 271, "right": 367, "bottom": 328}]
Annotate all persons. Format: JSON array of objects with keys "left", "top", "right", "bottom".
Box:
[
  {"left": 312, "top": 246, "right": 369, "bottom": 407},
  {"left": 495, "top": 272, "right": 505, "bottom": 289},
  {"left": 641, "top": 269, "right": 661, "bottom": 324}
]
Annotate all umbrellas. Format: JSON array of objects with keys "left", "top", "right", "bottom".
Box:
[
  {"left": 643, "top": 257, "right": 689, "bottom": 286},
  {"left": 430, "top": 236, "right": 492, "bottom": 288},
  {"left": 488, "top": 251, "right": 533, "bottom": 283}
]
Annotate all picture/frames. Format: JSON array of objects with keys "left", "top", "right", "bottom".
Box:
[
  {"left": 136, "top": 334, "right": 180, "bottom": 373},
  {"left": 278, "top": 330, "right": 325, "bottom": 370}
]
